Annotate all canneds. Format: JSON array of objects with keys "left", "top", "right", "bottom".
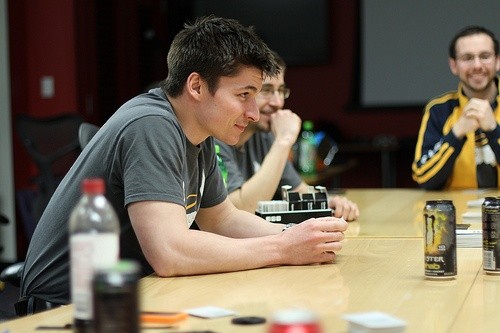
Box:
[
  {"left": 481, "top": 195, "right": 500, "bottom": 275},
  {"left": 269, "top": 309, "right": 322, "bottom": 333},
  {"left": 422, "top": 200, "right": 458, "bottom": 280},
  {"left": 91, "top": 260, "right": 142, "bottom": 333}
]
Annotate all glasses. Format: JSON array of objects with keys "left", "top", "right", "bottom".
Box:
[{"left": 258, "top": 86, "right": 291, "bottom": 100}]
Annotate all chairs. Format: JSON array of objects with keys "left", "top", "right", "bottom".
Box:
[{"left": 15, "top": 112, "right": 81, "bottom": 249}]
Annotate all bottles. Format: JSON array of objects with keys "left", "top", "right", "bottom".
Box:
[
  {"left": 297, "top": 121, "right": 320, "bottom": 186},
  {"left": 211, "top": 143, "right": 229, "bottom": 195},
  {"left": 67, "top": 178, "right": 120, "bottom": 332}
]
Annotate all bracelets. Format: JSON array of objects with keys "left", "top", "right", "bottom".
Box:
[{"left": 282, "top": 223, "right": 294, "bottom": 231}]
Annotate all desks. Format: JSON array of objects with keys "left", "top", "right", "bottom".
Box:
[
  {"left": 300, "top": 136, "right": 400, "bottom": 184},
  {"left": 0, "top": 186, "right": 500, "bottom": 333}
]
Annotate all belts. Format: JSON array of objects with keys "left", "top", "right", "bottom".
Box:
[{"left": 14, "top": 297, "right": 63, "bottom": 316}]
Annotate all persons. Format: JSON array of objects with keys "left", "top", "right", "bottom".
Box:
[
  {"left": 14, "top": 18, "right": 348, "bottom": 316},
  {"left": 214, "top": 52, "right": 358, "bottom": 222},
  {"left": 411, "top": 26, "right": 500, "bottom": 190}
]
